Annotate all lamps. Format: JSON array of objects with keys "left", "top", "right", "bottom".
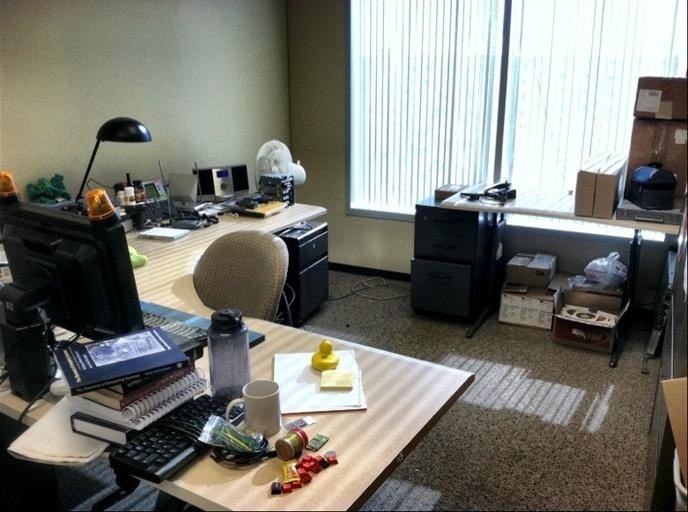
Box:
[{"left": 69, "top": 113, "right": 152, "bottom": 206}]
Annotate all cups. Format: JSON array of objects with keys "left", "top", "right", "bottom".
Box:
[{"left": 224, "top": 380, "right": 284, "bottom": 439}]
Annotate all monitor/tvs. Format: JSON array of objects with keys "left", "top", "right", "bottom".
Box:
[{"left": 0, "top": 195, "right": 144, "bottom": 403}]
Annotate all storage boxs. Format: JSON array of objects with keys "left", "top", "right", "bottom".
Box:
[
  {"left": 496, "top": 238, "right": 636, "bottom": 359},
  {"left": 567, "top": 67, "right": 687, "bottom": 224}
]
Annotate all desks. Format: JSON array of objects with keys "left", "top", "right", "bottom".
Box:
[
  {"left": 1, "top": 176, "right": 478, "bottom": 511},
  {"left": 439, "top": 179, "right": 685, "bottom": 373}
]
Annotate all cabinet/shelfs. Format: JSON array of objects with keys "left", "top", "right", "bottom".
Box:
[
  {"left": 405, "top": 186, "right": 510, "bottom": 327},
  {"left": 644, "top": 207, "right": 688, "bottom": 512},
  {"left": 273, "top": 218, "right": 334, "bottom": 329}
]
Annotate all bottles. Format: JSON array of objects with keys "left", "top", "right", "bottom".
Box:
[
  {"left": 132, "top": 179, "right": 146, "bottom": 203},
  {"left": 124, "top": 187, "right": 136, "bottom": 204},
  {"left": 206, "top": 307, "right": 250, "bottom": 399},
  {"left": 115, "top": 191, "right": 126, "bottom": 206},
  {"left": 274, "top": 427, "right": 310, "bottom": 460}
]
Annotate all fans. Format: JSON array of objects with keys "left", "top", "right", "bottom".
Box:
[{"left": 246, "top": 138, "right": 308, "bottom": 205}]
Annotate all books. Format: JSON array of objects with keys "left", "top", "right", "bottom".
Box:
[
  {"left": 220, "top": 198, "right": 288, "bottom": 219},
  {"left": 137, "top": 226, "right": 190, "bottom": 241},
  {"left": 0, "top": 325, "right": 207, "bottom": 468}
]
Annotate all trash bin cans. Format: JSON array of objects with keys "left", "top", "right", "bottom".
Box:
[{"left": 673, "top": 448, "right": 688, "bottom": 511}]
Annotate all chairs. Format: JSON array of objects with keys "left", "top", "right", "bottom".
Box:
[{"left": 193, "top": 226, "right": 298, "bottom": 330}]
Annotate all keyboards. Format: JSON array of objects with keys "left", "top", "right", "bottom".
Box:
[
  {"left": 109, "top": 393, "right": 244, "bottom": 484},
  {"left": 141, "top": 311, "right": 209, "bottom": 346}
]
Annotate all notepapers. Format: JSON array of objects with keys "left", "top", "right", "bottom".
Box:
[{"left": 320, "top": 370, "right": 355, "bottom": 391}]
